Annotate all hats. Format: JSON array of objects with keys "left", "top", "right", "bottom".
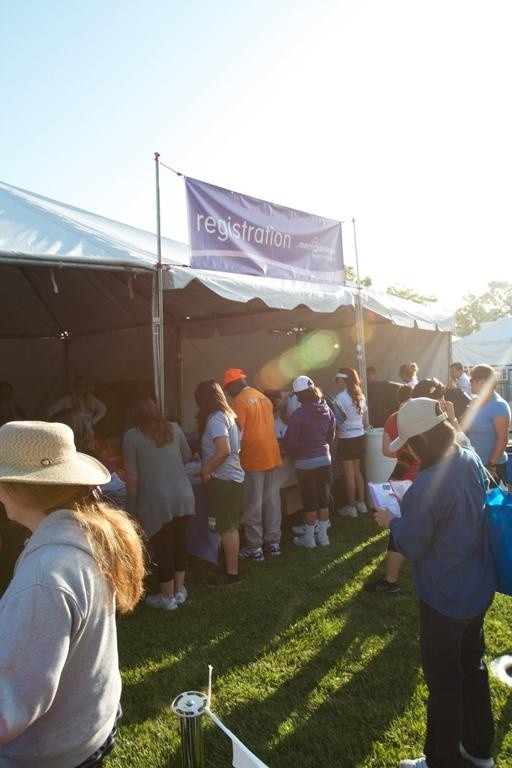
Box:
[
  {"left": 288, "top": 376, "right": 315, "bottom": 398},
  {"left": 0, "top": 421, "right": 111, "bottom": 487},
  {"left": 220, "top": 367, "right": 246, "bottom": 390},
  {"left": 387, "top": 397, "right": 449, "bottom": 452}
]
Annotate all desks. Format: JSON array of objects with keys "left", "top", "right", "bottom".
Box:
[
  {"left": 277, "top": 451, "right": 299, "bottom": 490},
  {"left": 98, "top": 471, "right": 215, "bottom": 565}
]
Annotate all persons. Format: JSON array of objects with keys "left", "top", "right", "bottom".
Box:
[
  {"left": 0, "top": 359, "right": 369, "bottom": 613},
  {"left": 372, "top": 397, "right": 498, "bottom": 768},
  {"left": 365, "top": 361, "right": 510, "bottom": 594},
  {"left": 0, "top": 421, "right": 146, "bottom": 767}
]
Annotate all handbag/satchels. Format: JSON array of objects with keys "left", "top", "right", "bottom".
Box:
[
  {"left": 183, "top": 516, "right": 222, "bottom": 565},
  {"left": 485, "top": 486, "right": 512, "bottom": 596}
]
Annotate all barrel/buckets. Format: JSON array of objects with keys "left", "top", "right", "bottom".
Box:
[{"left": 362, "top": 428, "right": 399, "bottom": 510}]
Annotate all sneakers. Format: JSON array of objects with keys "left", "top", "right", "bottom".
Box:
[
  {"left": 364, "top": 576, "right": 401, "bottom": 595},
  {"left": 292, "top": 517, "right": 332, "bottom": 548},
  {"left": 210, "top": 543, "right": 282, "bottom": 587},
  {"left": 144, "top": 584, "right": 188, "bottom": 610},
  {"left": 338, "top": 501, "right": 368, "bottom": 517}
]
